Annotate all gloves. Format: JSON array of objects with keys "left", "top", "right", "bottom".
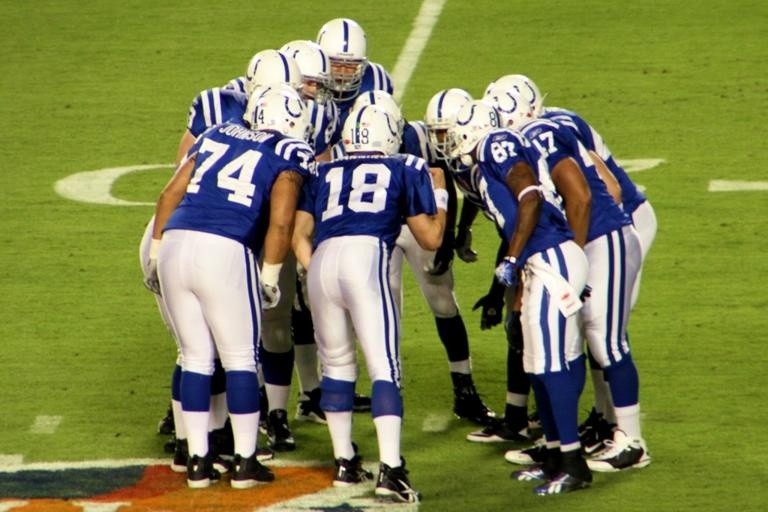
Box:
[
  {"left": 424, "top": 250, "right": 452, "bottom": 276},
  {"left": 454, "top": 229, "right": 478, "bottom": 264},
  {"left": 142, "top": 239, "right": 160, "bottom": 295},
  {"left": 260, "top": 262, "right": 283, "bottom": 309},
  {"left": 492, "top": 259, "right": 518, "bottom": 287},
  {"left": 473, "top": 294, "right": 503, "bottom": 330}
]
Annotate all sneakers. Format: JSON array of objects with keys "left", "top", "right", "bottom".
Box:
[
  {"left": 452, "top": 390, "right": 649, "bottom": 495},
  {"left": 296, "top": 388, "right": 328, "bottom": 425},
  {"left": 375, "top": 457, "right": 421, "bottom": 502},
  {"left": 353, "top": 391, "right": 372, "bottom": 413},
  {"left": 332, "top": 444, "right": 372, "bottom": 486},
  {"left": 158, "top": 384, "right": 295, "bottom": 490}
]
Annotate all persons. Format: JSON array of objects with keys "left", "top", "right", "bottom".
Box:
[
  {"left": 446, "top": 99, "right": 593, "bottom": 498},
  {"left": 137, "top": 17, "right": 659, "bottom": 475},
  {"left": 142, "top": 93, "right": 317, "bottom": 490},
  {"left": 288, "top": 103, "right": 450, "bottom": 504}
]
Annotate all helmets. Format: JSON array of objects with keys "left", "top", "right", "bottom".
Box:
[
  {"left": 244, "top": 19, "right": 405, "bottom": 156},
  {"left": 426, "top": 74, "right": 544, "bottom": 173}
]
